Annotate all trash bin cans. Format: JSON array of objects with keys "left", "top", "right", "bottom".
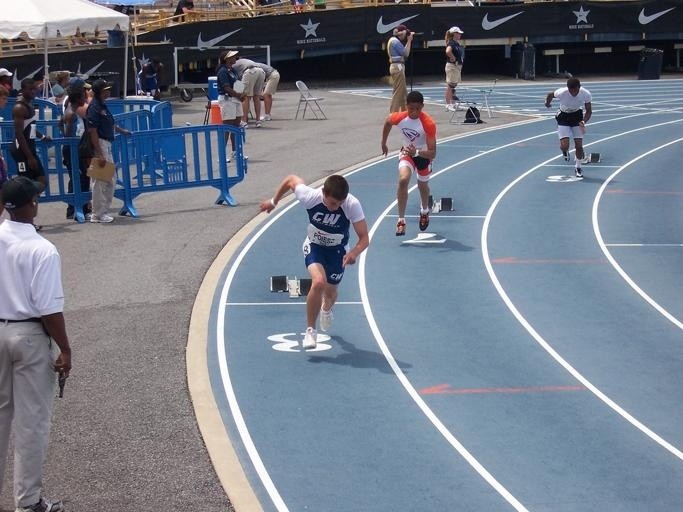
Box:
[
  {"left": 88, "top": 72, "right": 122, "bottom": 97},
  {"left": 637, "top": 48, "right": 664, "bottom": 80}
]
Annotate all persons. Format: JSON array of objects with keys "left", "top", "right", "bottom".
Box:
[
  {"left": 445, "top": 27, "right": 464, "bottom": 112},
  {"left": 0, "top": 177, "right": 71, "bottom": 512},
  {"left": 544, "top": 79, "right": 592, "bottom": 180},
  {"left": 381, "top": 91, "right": 436, "bottom": 236},
  {"left": 136, "top": 62, "right": 158, "bottom": 96},
  {"left": 215, "top": 50, "right": 249, "bottom": 162},
  {"left": 256, "top": 62, "right": 280, "bottom": 121},
  {"left": 387, "top": 24, "right": 415, "bottom": 113},
  {"left": 0, "top": 68, "right": 132, "bottom": 232},
  {"left": 169, "top": 0, "right": 194, "bottom": 27},
  {"left": 232, "top": 58, "right": 266, "bottom": 128},
  {"left": 259, "top": 174, "right": 369, "bottom": 349}
]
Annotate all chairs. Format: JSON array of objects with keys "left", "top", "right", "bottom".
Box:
[
  {"left": 444, "top": 84, "right": 480, "bottom": 122},
  {"left": 293, "top": 80, "right": 329, "bottom": 121},
  {"left": 474, "top": 78, "right": 500, "bottom": 118}
]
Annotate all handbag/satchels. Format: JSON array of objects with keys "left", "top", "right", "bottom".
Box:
[{"left": 77, "top": 131, "right": 93, "bottom": 158}]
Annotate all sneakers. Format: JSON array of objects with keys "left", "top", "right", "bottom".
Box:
[
  {"left": 419, "top": 213, "right": 428, "bottom": 230},
  {"left": 396, "top": 221, "right": 405, "bottom": 235},
  {"left": 302, "top": 327, "right": 316, "bottom": 348},
  {"left": 85, "top": 214, "right": 113, "bottom": 222},
  {"left": 16, "top": 497, "right": 63, "bottom": 512},
  {"left": 444, "top": 104, "right": 462, "bottom": 112},
  {"left": 575, "top": 168, "right": 582, "bottom": 177},
  {"left": 240, "top": 114, "right": 270, "bottom": 128},
  {"left": 563, "top": 151, "right": 569, "bottom": 161},
  {"left": 320, "top": 309, "right": 331, "bottom": 331},
  {"left": 229, "top": 151, "right": 247, "bottom": 160}
]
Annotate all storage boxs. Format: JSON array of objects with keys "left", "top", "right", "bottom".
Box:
[{"left": 211, "top": 100, "right": 223, "bottom": 126}]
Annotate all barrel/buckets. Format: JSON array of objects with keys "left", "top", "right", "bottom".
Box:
[
  {"left": 210, "top": 100, "right": 223, "bottom": 124},
  {"left": 106, "top": 30, "right": 125, "bottom": 49}
]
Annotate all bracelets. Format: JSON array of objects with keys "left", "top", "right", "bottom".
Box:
[
  {"left": 271, "top": 198, "right": 276, "bottom": 207},
  {"left": 581, "top": 120, "right": 585, "bottom": 125},
  {"left": 414, "top": 150, "right": 419, "bottom": 157}
]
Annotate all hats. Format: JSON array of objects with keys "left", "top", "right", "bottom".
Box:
[
  {"left": 92, "top": 79, "right": 111, "bottom": 92},
  {"left": 69, "top": 78, "right": 91, "bottom": 88},
  {"left": 223, "top": 50, "right": 239, "bottom": 59},
  {"left": 0, "top": 177, "right": 44, "bottom": 208},
  {"left": 449, "top": 27, "right": 463, "bottom": 34},
  {"left": 0, "top": 68, "right": 12, "bottom": 77}
]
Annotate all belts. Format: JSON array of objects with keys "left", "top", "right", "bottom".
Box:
[{"left": 0, "top": 318, "right": 41, "bottom": 322}]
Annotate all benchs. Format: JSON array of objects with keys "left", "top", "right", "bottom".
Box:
[{"left": 0, "top": 0, "right": 432, "bottom": 48}]
